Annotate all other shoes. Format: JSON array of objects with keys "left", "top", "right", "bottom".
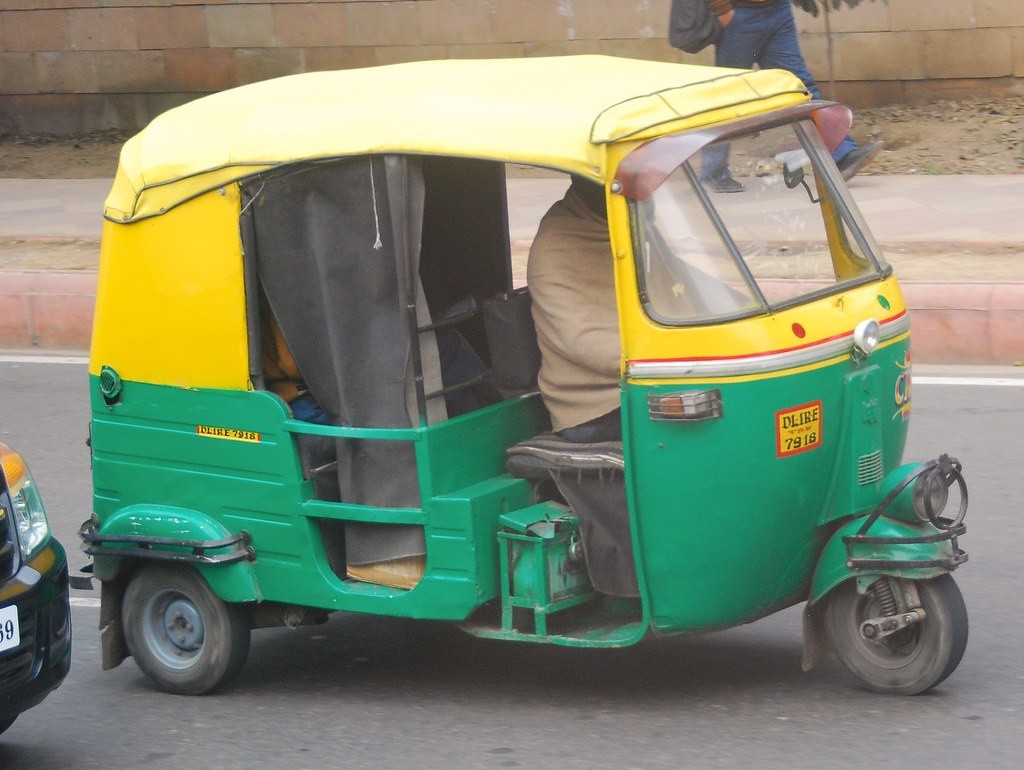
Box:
[
  {"left": 703, "top": 167, "right": 746, "bottom": 194},
  {"left": 837, "top": 139, "right": 886, "bottom": 182}
]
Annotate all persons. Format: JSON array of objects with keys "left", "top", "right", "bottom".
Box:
[
  {"left": 256, "top": 285, "right": 335, "bottom": 425},
  {"left": 527, "top": 170, "right": 718, "bottom": 444},
  {"left": 668, "top": 1, "right": 883, "bottom": 194}
]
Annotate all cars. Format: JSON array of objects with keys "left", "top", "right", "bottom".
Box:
[{"left": 0, "top": 432, "right": 74, "bottom": 740}]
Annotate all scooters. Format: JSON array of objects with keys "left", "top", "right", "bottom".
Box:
[{"left": 63, "top": 51, "right": 971, "bottom": 697}]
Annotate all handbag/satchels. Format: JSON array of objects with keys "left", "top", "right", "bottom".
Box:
[{"left": 667, "top": 0, "right": 722, "bottom": 55}]
